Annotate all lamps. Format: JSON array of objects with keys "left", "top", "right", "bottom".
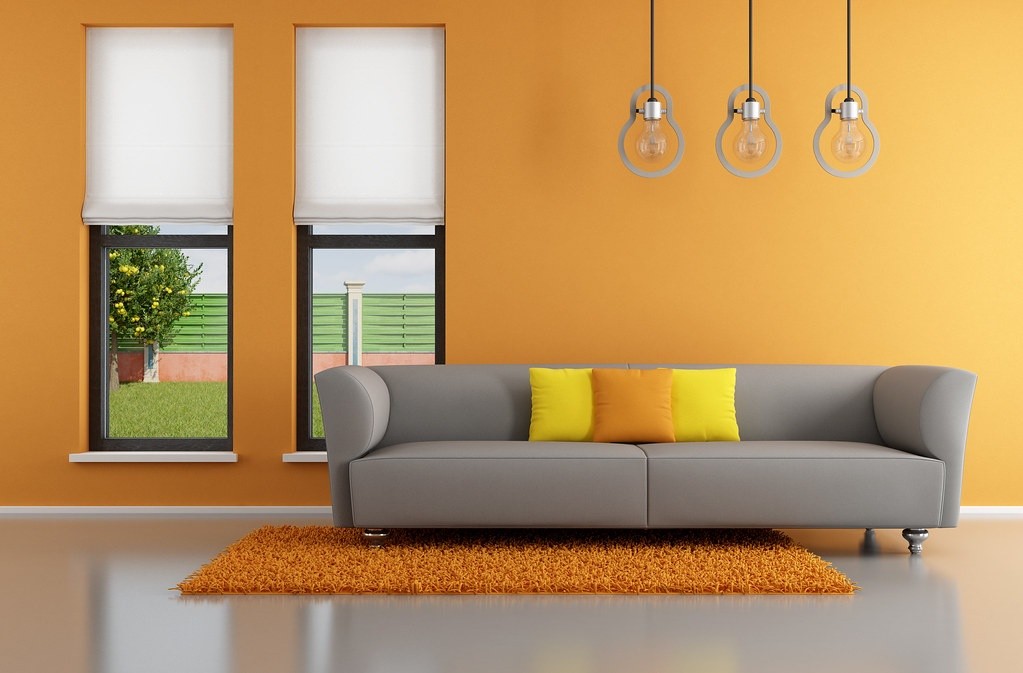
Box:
[
  {"left": 813, "top": 0, "right": 880, "bottom": 178},
  {"left": 618, "top": 0, "right": 686, "bottom": 178},
  {"left": 717, "top": 0, "right": 781, "bottom": 178}
]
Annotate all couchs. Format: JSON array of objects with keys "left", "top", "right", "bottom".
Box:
[{"left": 313, "top": 364, "right": 977, "bottom": 552}]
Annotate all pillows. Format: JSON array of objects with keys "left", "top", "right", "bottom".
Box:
[
  {"left": 590, "top": 369, "right": 676, "bottom": 443},
  {"left": 526, "top": 367, "right": 594, "bottom": 441},
  {"left": 672, "top": 368, "right": 741, "bottom": 443}
]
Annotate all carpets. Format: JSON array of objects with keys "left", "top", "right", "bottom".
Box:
[{"left": 181, "top": 523, "right": 859, "bottom": 599}]
